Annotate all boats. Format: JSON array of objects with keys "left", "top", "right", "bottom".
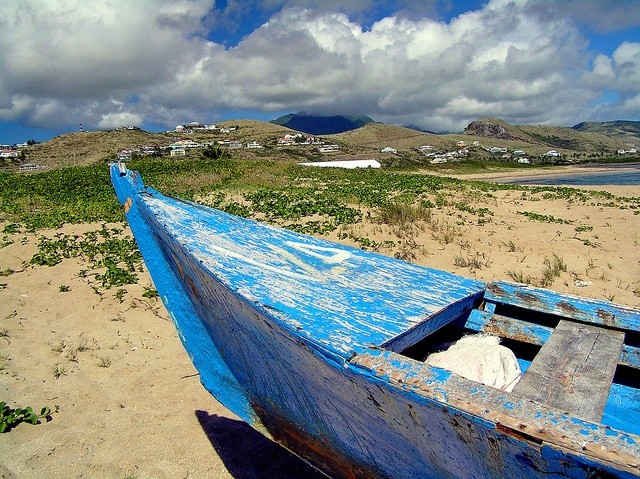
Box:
[{"left": 110, "top": 162, "right": 640, "bottom": 478}]
